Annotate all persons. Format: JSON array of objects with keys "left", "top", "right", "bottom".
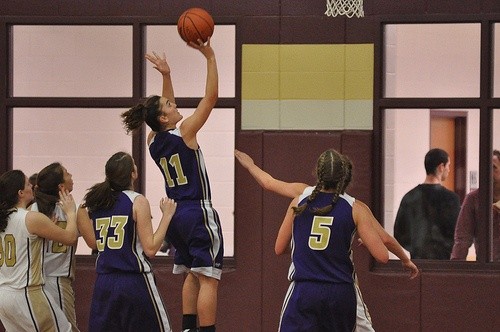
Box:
[
  {"left": 451, "top": 149, "right": 500, "bottom": 261},
  {"left": 393, "top": 148, "right": 461, "bottom": 261},
  {"left": 234, "top": 147, "right": 420, "bottom": 332},
  {"left": 81, "top": 151, "right": 178, "bottom": 332},
  {"left": 121, "top": 37, "right": 225, "bottom": 332},
  {"left": 0, "top": 162, "right": 97, "bottom": 332}
]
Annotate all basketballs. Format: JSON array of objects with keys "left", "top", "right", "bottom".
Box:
[{"left": 178, "top": 6, "right": 214, "bottom": 43}]
